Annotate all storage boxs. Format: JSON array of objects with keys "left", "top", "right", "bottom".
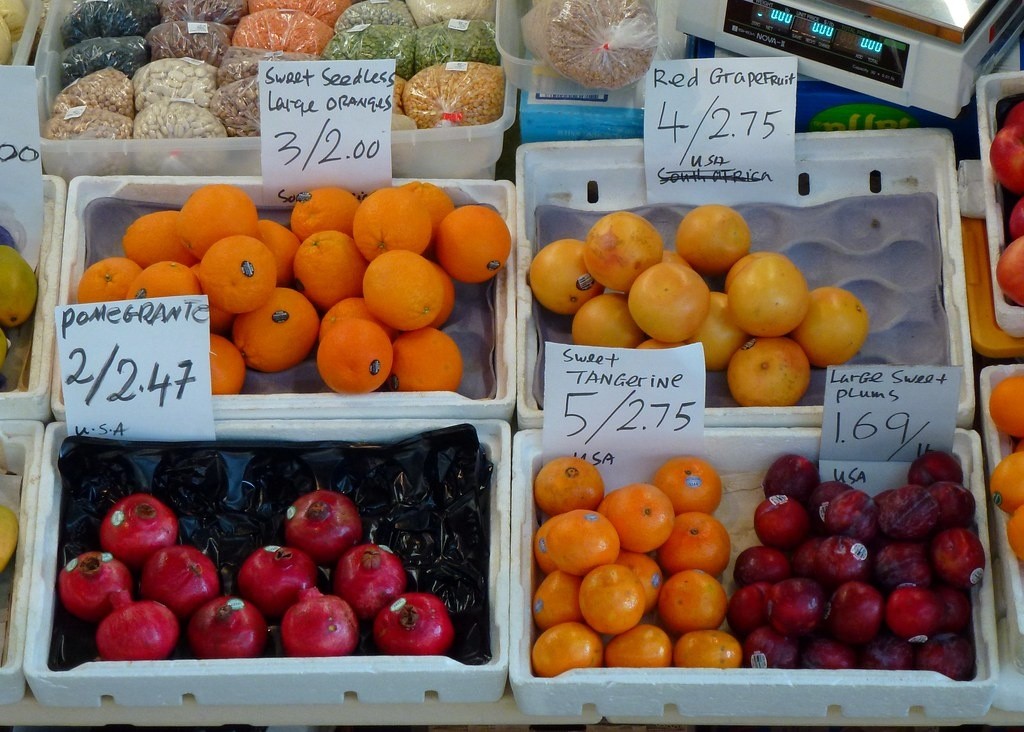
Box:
[{"left": 1, "top": 1, "right": 1022, "bottom": 727}]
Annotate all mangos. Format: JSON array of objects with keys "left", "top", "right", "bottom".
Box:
[{"left": 0, "top": 245, "right": 36, "bottom": 368}]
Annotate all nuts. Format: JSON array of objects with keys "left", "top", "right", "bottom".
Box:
[{"left": 44, "top": 1, "right": 507, "bottom": 141}]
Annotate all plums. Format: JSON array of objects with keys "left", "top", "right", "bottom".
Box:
[{"left": 729, "top": 450, "right": 987, "bottom": 681}]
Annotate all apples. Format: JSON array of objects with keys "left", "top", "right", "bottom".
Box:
[{"left": 989, "top": 100, "right": 1024, "bottom": 307}]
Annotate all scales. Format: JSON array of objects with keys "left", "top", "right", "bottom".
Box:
[{"left": 676, "top": 0, "right": 1022, "bottom": 129}]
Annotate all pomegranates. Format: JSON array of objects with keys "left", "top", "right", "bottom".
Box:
[{"left": 59, "top": 491, "right": 454, "bottom": 661}]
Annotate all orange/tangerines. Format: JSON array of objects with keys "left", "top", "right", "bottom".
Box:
[
  {"left": 75, "top": 184, "right": 511, "bottom": 393},
  {"left": 531, "top": 456, "right": 740, "bottom": 676},
  {"left": 986, "top": 374, "right": 1024, "bottom": 554},
  {"left": 529, "top": 204, "right": 867, "bottom": 406}
]
